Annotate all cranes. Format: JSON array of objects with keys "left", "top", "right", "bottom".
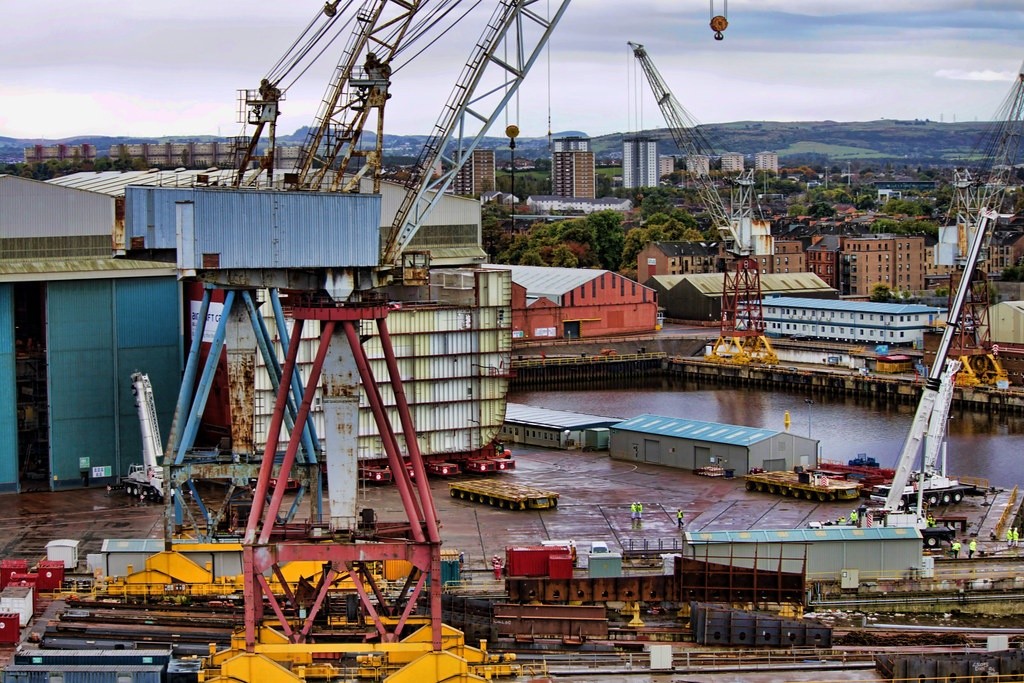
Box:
[{"left": 0, "top": 0, "right": 1024, "bottom": 680}]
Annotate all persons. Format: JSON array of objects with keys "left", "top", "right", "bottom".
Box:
[
  {"left": 676, "top": 508, "right": 684, "bottom": 528},
  {"left": 968, "top": 539, "right": 976, "bottom": 559},
  {"left": 630, "top": 502, "right": 637, "bottom": 520},
  {"left": 637, "top": 501, "right": 642, "bottom": 519},
  {"left": 927, "top": 514, "right": 937, "bottom": 527},
  {"left": 951, "top": 540, "right": 961, "bottom": 559},
  {"left": 1007, "top": 528, "right": 1013, "bottom": 547},
  {"left": 850, "top": 510, "right": 858, "bottom": 525},
  {"left": 840, "top": 516, "right": 846, "bottom": 523},
  {"left": 1012, "top": 527, "right": 1019, "bottom": 548}
]
